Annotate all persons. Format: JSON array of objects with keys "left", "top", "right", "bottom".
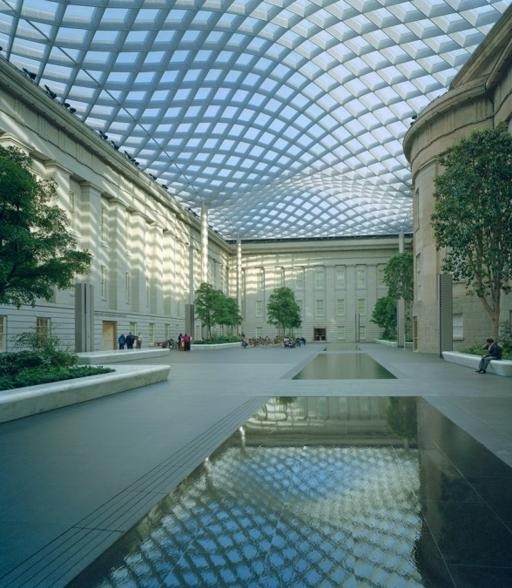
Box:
[
  {"left": 178, "top": 333, "right": 190, "bottom": 351},
  {"left": 118, "top": 332, "right": 142, "bottom": 349},
  {"left": 475, "top": 338, "right": 498, "bottom": 374}
]
